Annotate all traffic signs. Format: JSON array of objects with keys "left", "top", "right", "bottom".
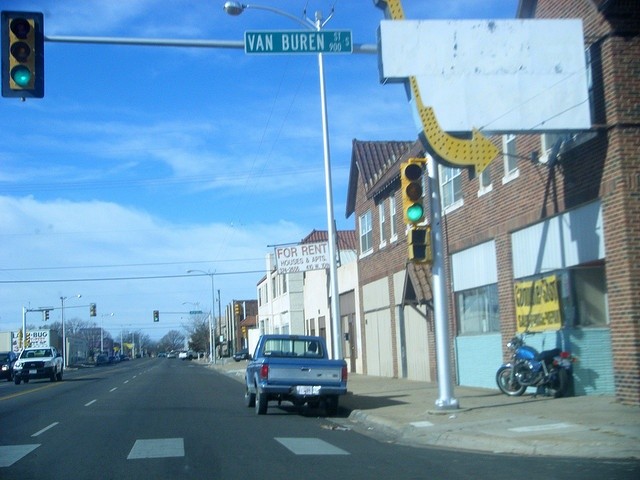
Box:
[
  {"left": 190, "top": 310, "right": 203, "bottom": 314},
  {"left": 244, "top": 29, "right": 353, "bottom": 55}
]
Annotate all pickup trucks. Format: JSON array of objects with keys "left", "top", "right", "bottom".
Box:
[{"left": 244, "top": 334, "right": 348, "bottom": 417}]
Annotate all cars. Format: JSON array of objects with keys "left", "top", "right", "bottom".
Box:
[
  {"left": 167, "top": 352, "right": 176, "bottom": 359},
  {"left": 1, "top": 351, "right": 18, "bottom": 382},
  {"left": 97, "top": 353, "right": 131, "bottom": 366},
  {"left": 232, "top": 348, "right": 249, "bottom": 362},
  {"left": 14, "top": 346, "right": 64, "bottom": 384},
  {"left": 179, "top": 350, "right": 203, "bottom": 360}
]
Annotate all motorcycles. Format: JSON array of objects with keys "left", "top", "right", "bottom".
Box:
[{"left": 498, "top": 330, "right": 578, "bottom": 398}]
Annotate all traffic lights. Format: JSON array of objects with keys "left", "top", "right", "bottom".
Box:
[
  {"left": 400, "top": 156, "right": 426, "bottom": 222},
  {"left": 90, "top": 303, "right": 97, "bottom": 316},
  {"left": 44, "top": 310, "right": 50, "bottom": 321},
  {"left": 0, "top": 10, "right": 44, "bottom": 99},
  {"left": 153, "top": 310, "right": 159, "bottom": 322},
  {"left": 406, "top": 227, "right": 432, "bottom": 266}
]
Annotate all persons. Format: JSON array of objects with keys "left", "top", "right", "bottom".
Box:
[{"left": 297, "top": 342, "right": 319, "bottom": 357}]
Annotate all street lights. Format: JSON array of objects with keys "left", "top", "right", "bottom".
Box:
[
  {"left": 101, "top": 312, "right": 114, "bottom": 352},
  {"left": 61, "top": 294, "right": 81, "bottom": 371},
  {"left": 224, "top": 2, "right": 343, "bottom": 361},
  {"left": 188, "top": 267, "right": 218, "bottom": 364}
]
